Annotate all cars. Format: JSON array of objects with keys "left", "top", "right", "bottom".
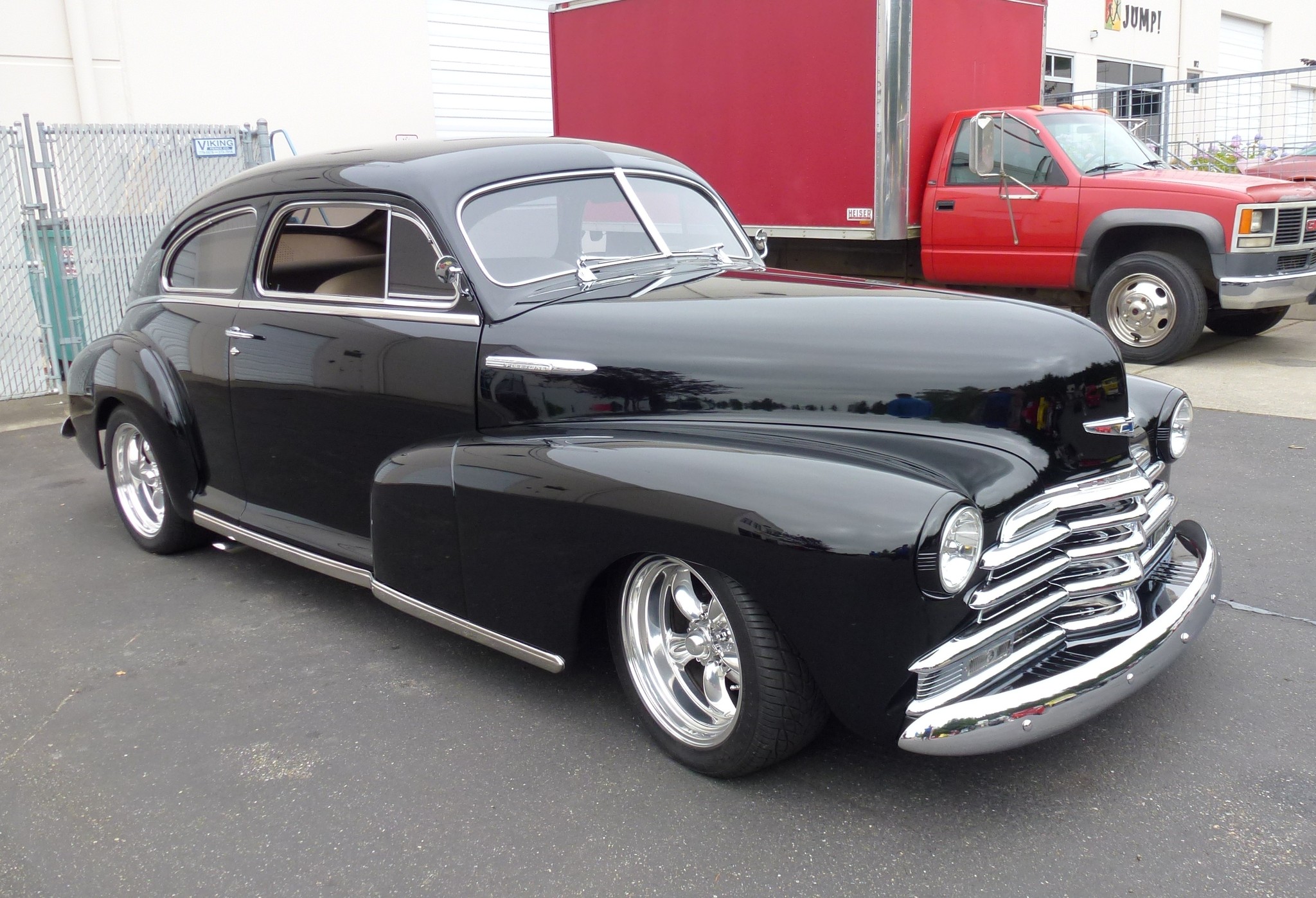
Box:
[
  {"left": 1244, "top": 142, "right": 1315, "bottom": 180},
  {"left": 65, "top": 137, "right": 1222, "bottom": 784}
]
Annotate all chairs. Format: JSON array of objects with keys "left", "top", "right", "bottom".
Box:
[
  {"left": 478, "top": 255, "right": 577, "bottom": 286},
  {"left": 308, "top": 257, "right": 458, "bottom": 298}
]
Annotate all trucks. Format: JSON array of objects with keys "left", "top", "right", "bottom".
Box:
[{"left": 545, "top": 1, "right": 1315, "bottom": 365}]
